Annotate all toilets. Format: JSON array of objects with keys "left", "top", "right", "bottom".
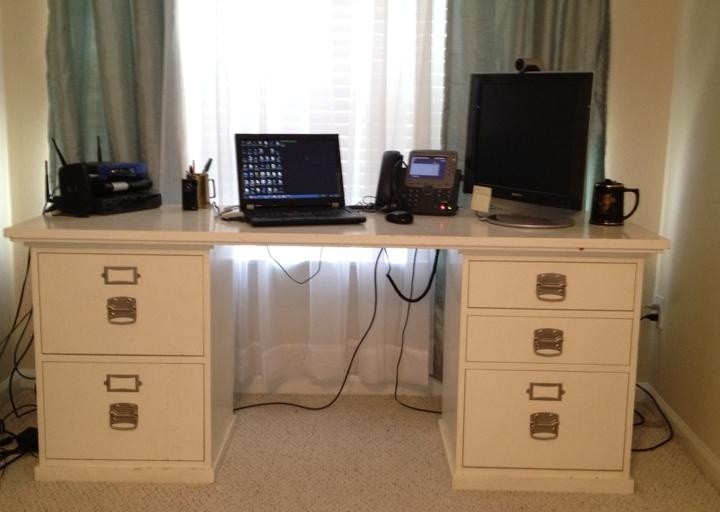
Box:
[{"left": 376, "top": 149, "right": 461, "bottom": 216}]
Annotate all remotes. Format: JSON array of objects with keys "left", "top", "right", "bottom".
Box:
[{"left": 385, "top": 210, "right": 414, "bottom": 224}]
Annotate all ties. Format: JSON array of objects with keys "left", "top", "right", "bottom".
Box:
[{"left": 462, "top": 71, "right": 594, "bottom": 230}]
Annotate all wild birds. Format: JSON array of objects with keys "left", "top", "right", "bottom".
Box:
[{"left": 234, "top": 133, "right": 367, "bottom": 226}]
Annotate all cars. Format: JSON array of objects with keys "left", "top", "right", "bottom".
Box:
[
  {"left": 589, "top": 179, "right": 639, "bottom": 225},
  {"left": 186, "top": 173, "right": 216, "bottom": 209}
]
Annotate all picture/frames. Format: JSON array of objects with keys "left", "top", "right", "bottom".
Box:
[{"left": 0, "top": 203, "right": 672, "bottom": 498}]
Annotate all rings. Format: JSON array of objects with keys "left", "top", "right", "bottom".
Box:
[{"left": 185, "top": 158, "right": 212, "bottom": 176}]
